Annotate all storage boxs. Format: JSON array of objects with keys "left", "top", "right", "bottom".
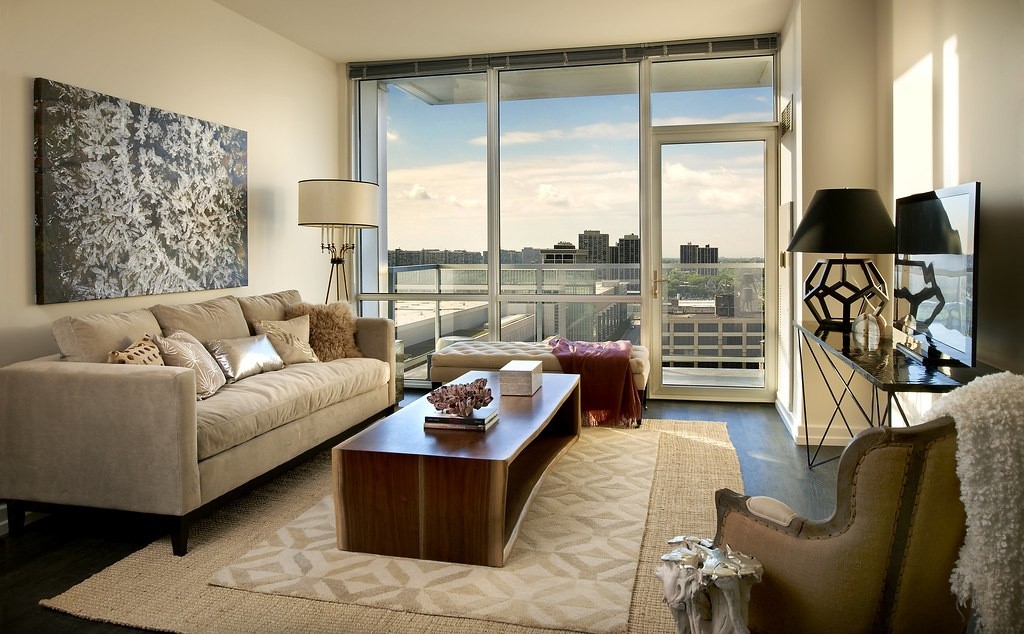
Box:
[{"left": 500, "top": 360, "right": 542, "bottom": 395}]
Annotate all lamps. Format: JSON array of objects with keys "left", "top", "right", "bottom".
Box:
[
  {"left": 787, "top": 189, "right": 895, "bottom": 330},
  {"left": 895, "top": 191, "right": 960, "bottom": 336},
  {"left": 298, "top": 179, "right": 379, "bottom": 305}
]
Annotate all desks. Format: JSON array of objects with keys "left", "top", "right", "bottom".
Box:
[
  {"left": 332, "top": 370, "right": 581, "bottom": 567},
  {"left": 791, "top": 320, "right": 1008, "bottom": 469}
]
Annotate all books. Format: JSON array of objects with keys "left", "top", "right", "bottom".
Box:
[
  {"left": 425, "top": 407, "right": 498, "bottom": 424},
  {"left": 424, "top": 414, "right": 500, "bottom": 431}
]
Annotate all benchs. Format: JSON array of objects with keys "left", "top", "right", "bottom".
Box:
[{"left": 431, "top": 342, "right": 650, "bottom": 429}]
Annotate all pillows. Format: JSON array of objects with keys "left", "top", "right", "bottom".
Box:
[{"left": 50, "top": 289, "right": 365, "bottom": 401}]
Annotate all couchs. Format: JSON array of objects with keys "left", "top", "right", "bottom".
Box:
[
  {"left": 0, "top": 290, "right": 395, "bottom": 556},
  {"left": 713, "top": 372, "right": 1024, "bottom": 634}
]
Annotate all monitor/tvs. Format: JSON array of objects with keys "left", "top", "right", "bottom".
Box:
[{"left": 893, "top": 181, "right": 981, "bottom": 367}]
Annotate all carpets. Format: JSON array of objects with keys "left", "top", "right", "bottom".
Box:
[
  {"left": 206, "top": 428, "right": 660, "bottom": 634},
  {"left": 38, "top": 405, "right": 745, "bottom": 634}
]
